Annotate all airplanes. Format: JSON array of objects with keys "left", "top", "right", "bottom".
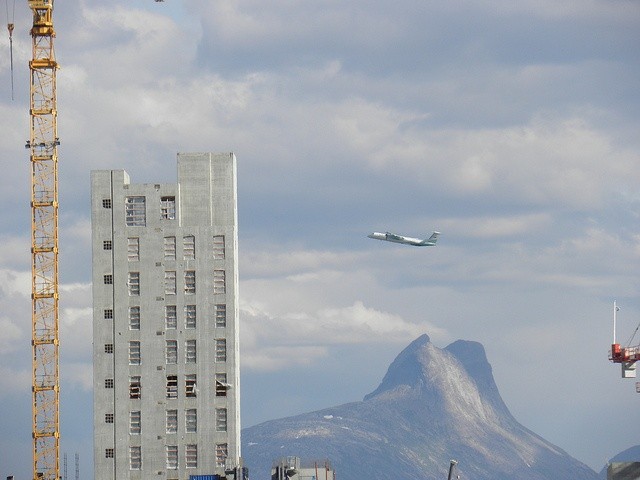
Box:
[{"left": 368, "top": 232, "right": 440, "bottom": 246}]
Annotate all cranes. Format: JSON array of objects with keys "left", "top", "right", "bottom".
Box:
[
  {"left": 607, "top": 301, "right": 640, "bottom": 394},
  {"left": 6, "top": 0, "right": 59, "bottom": 480}
]
